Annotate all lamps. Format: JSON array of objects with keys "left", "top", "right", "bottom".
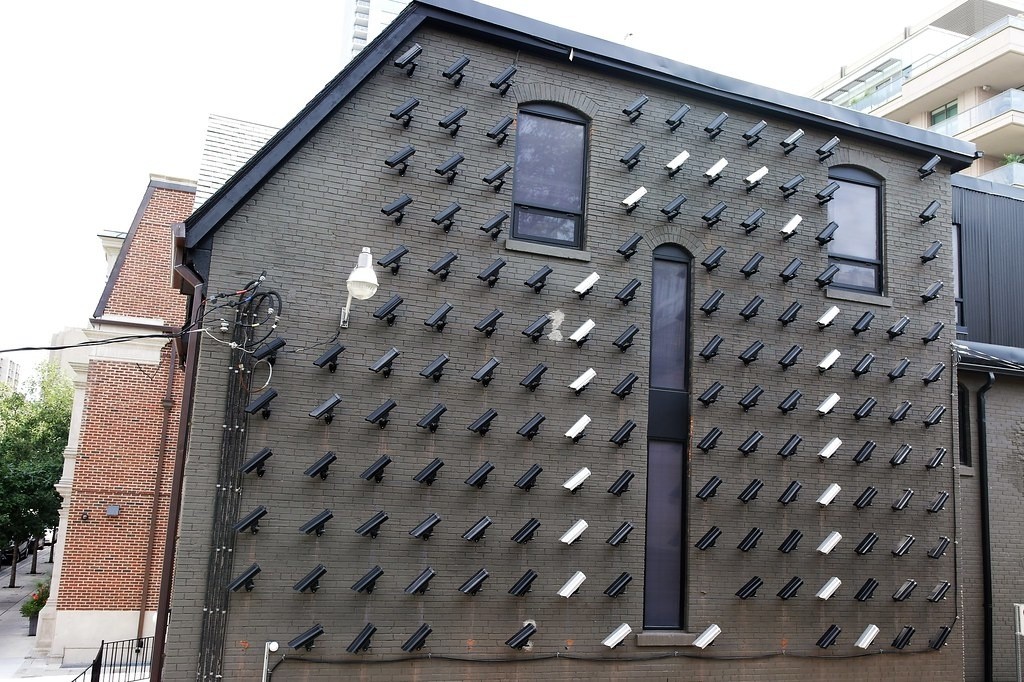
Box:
[{"left": 338, "top": 246, "right": 381, "bottom": 330}]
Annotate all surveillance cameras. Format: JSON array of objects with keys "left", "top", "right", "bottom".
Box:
[{"left": 227, "top": 42, "right": 951, "bottom": 655}]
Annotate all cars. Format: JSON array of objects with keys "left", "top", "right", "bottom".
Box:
[
  {"left": 44, "top": 527, "right": 58, "bottom": 545},
  {"left": 29, "top": 535, "right": 46, "bottom": 550},
  {"left": 0, "top": 538, "right": 29, "bottom": 564}
]
